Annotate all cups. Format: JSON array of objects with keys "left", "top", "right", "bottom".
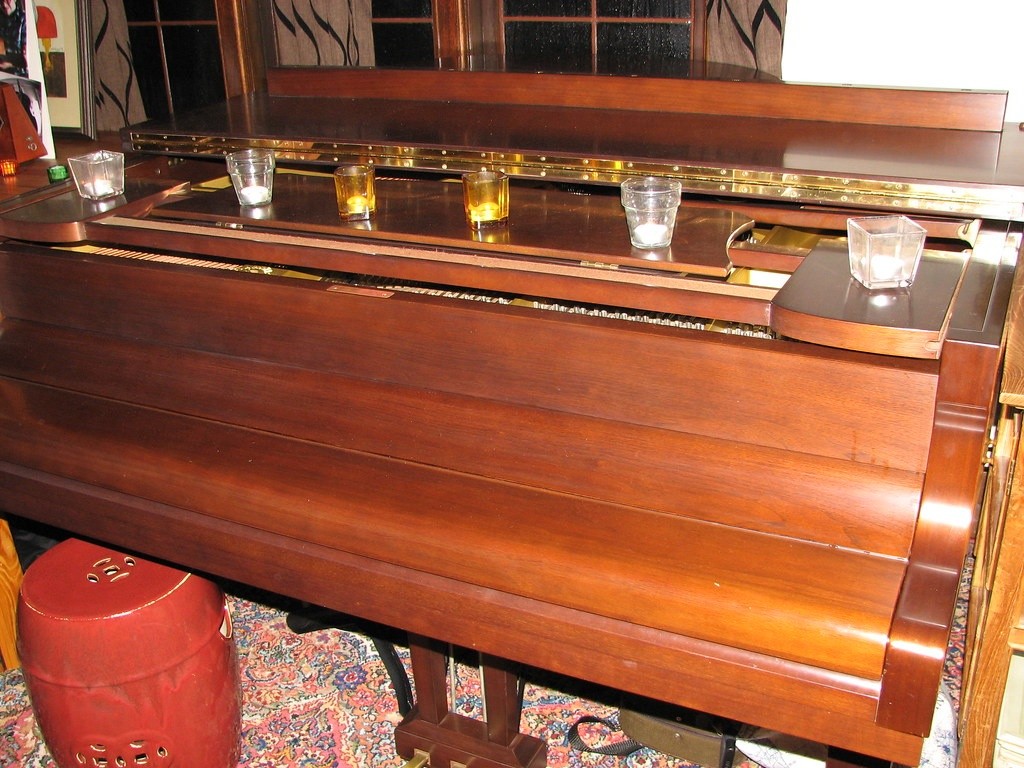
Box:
[
  {"left": 462, "top": 171, "right": 510, "bottom": 231},
  {"left": 67, "top": 149, "right": 125, "bottom": 200},
  {"left": 846, "top": 215, "right": 927, "bottom": 290},
  {"left": 225, "top": 148, "right": 276, "bottom": 206},
  {"left": 620, "top": 176, "right": 682, "bottom": 250},
  {"left": 333, "top": 164, "right": 377, "bottom": 221}
]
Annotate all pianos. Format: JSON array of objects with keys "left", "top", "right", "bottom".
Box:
[{"left": 0, "top": 64, "right": 1024, "bottom": 767}]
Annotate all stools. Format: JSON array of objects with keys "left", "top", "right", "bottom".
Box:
[{"left": 14, "top": 538, "right": 241, "bottom": 768}]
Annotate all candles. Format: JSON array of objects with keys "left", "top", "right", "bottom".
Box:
[
  {"left": 634, "top": 223, "right": 669, "bottom": 245},
  {"left": 473, "top": 202, "right": 500, "bottom": 219},
  {"left": 84, "top": 180, "right": 113, "bottom": 194},
  {"left": 240, "top": 184, "right": 269, "bottom": 202},
  {"left": 863, "top": 254, "right": 905, "bottom": 280},
  {"left": 345, "top": 190, "right": 371, "bottom": 212}
]
